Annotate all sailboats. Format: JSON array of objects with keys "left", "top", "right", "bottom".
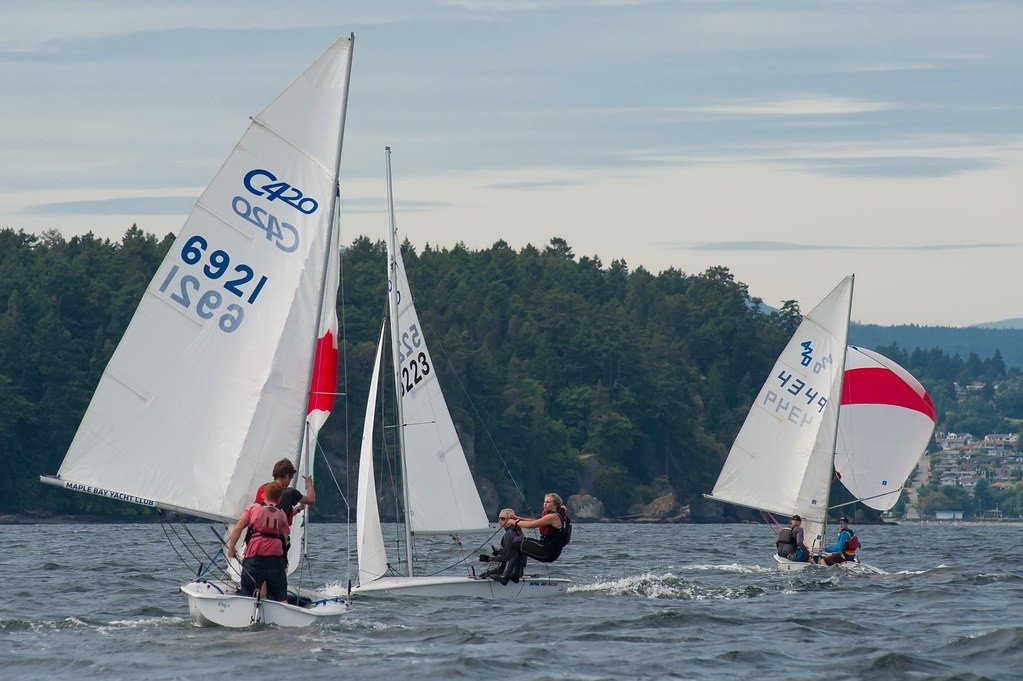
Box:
[
  {"left": 702, "top": 274, "right": 937, "bottom": 573},
  {"left": 39, "top": 33, "right": 351, "bottom": 629},
  {"left": 349, "top": 145, "right": 573, "bottom": 601}
]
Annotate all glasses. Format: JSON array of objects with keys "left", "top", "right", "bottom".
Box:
[
  {"left": 498, "top": 517, "right": 513, "bottom": 520},
  {"left": 838, "top": 522, "right": 846, "bottom": 525}
]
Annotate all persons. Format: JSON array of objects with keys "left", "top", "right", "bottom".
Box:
[
  {"left": 254, "top": 458, "right": 316, "bottom": 599},
  {"left": 489, "top": 493, "right": 572, "bottom": 587},
  {"left": 473, "top": 508, "right": 527, "bottom": 581},
  {"left": 775, "top": 515, "right": 813, "bottom": 563},
  {"left": 227, "top": 482, "right": 290, "bottom": 605},
  {"left": 819, "top": 517, "right": 862, "bottom": 567}
]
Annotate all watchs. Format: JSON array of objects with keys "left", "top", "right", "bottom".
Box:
[{"left": 514, "top": 519, "right": 520, "bottom": 527}]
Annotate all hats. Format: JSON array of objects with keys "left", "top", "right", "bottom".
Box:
[
  {"left": 838, "top": 517, "right": 849, "bottom": 523},
  {"left": 790, "top": 515, "right": 802, "bottom": 521}
]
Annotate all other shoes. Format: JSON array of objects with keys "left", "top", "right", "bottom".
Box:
[
  {"left": 489, "top": 574, "right": 508, "bottom": 586},
  {"left": 508, "top": 573, "right": 519, "bottom": 583}
]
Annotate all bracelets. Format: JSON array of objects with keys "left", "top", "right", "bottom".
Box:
[{"left": 294, "top": 506, "right": 300, "bottom": 513}]
